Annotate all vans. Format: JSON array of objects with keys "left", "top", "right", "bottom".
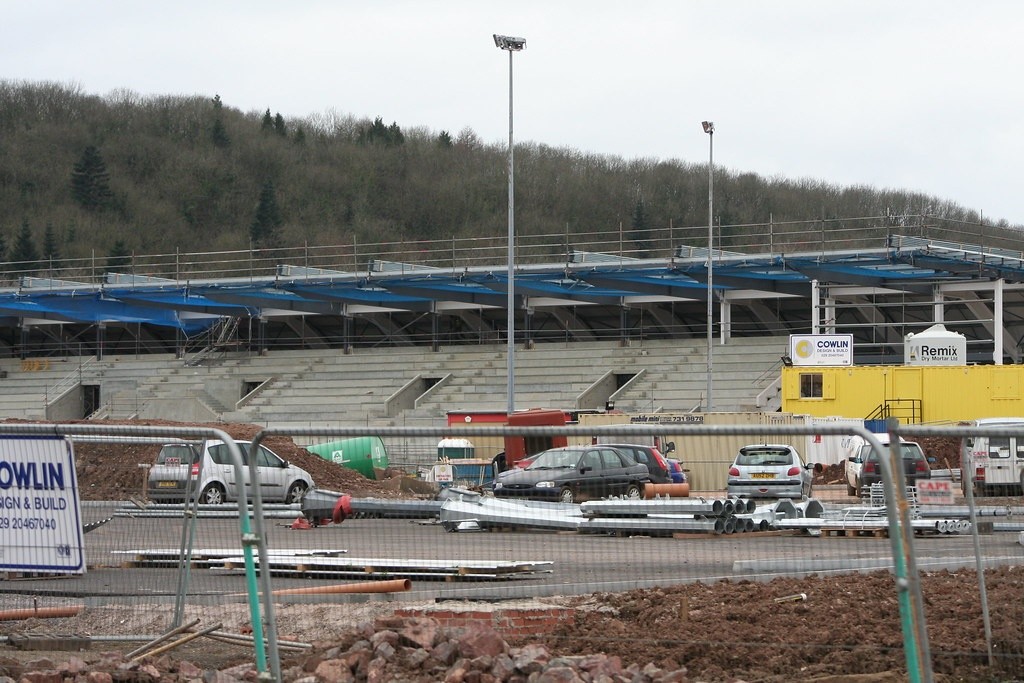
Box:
[{"left": 959, "top": 417, "right": 1024, "bottom": 495}]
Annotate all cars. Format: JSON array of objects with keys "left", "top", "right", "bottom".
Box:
[
  {"left": 845, "top": 433, "right": 936, "bottom": 497},
  {"left": 727, "top": 444, "right": 815, "bottom": 502},
  {"left": 492, "top": 442, "right": 690, "bottom": 503},
  {"left": 145, "top": 440, "right": 315, "bottom": 504}
]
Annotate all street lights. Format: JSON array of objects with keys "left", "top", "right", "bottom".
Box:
[
  {"left": 491, "top": 31, "right": 528, "bottom": 417},
  {"left": 701, "top": 118, "right": 721, "bottom": 416}
]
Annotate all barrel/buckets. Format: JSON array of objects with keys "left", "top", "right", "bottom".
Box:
[{"left": 305, "top": 436, "right": 388, "bottom": 479}]
